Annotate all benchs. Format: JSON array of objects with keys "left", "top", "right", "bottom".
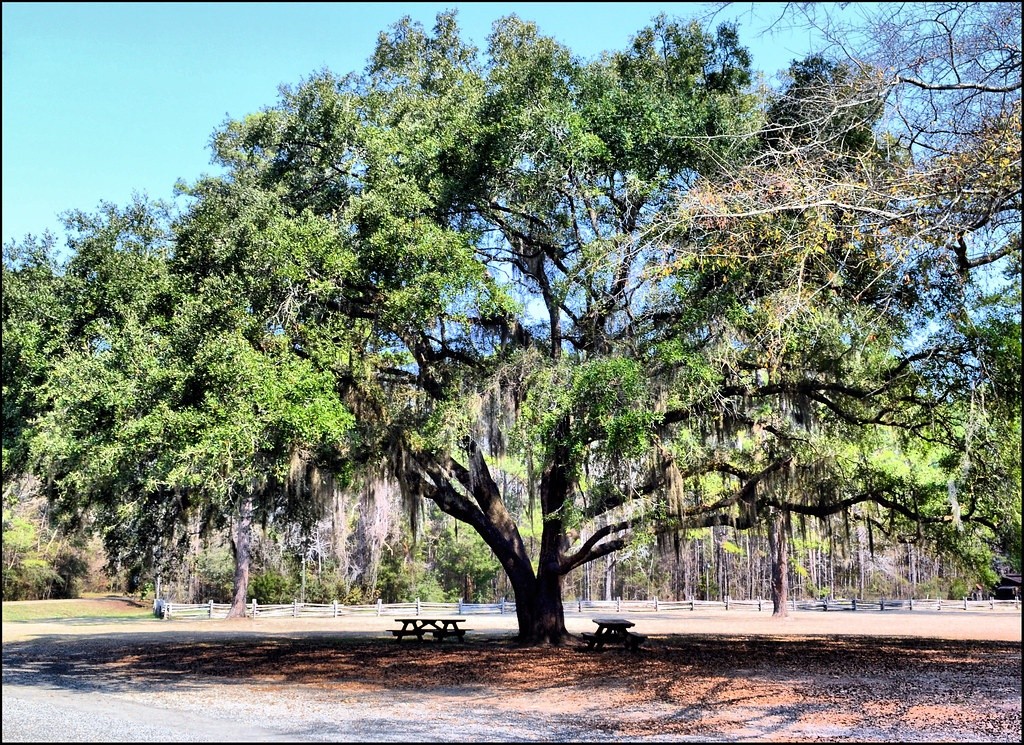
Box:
[
  {"left": 386, "top": 629, "right": 474, "bottom": 643},
  {"left": 628, "top": 631, "right": 647, "bottom": 653},
  {"left": 581, "top": 632, "right": 599, "bottom": 653}
]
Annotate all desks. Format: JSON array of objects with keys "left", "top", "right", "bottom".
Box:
[
  {"left": 586, "top": 619, "right": 639, "bottom": 653},
  {"left": 395, "top": 619, "right": 466, "bottom": 643}
]
